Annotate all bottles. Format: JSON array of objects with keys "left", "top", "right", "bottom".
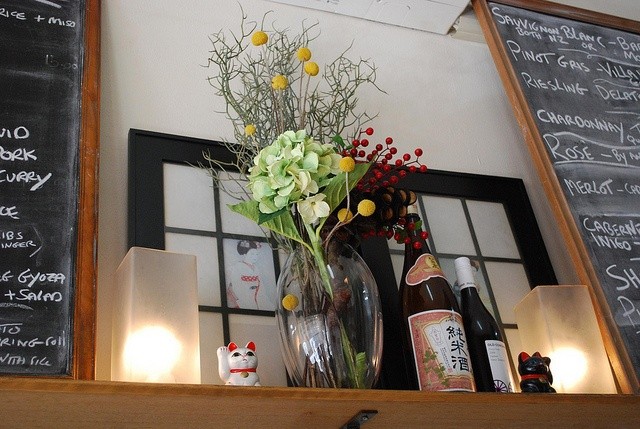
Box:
[
  {"left": 453, "top": 256, "right": 513, "bottom": 394},
  {"left": 397, "top": 212, "right": 476, "bottom": 393}
]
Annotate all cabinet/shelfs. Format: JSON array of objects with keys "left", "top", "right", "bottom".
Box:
[{"left": 0, "top": 378, "right": 637, "bottom": 429}]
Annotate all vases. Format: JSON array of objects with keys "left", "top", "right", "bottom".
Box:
[{"left": 271, "top": 235, "right": 384, "bottom": 388}]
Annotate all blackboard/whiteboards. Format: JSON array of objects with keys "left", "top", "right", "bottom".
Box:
[
  {"left": 487, "top": 0, "right": 640, "bottom": 396},
  {"left": 0, "top": 0, "right": 86, "bottom": 378}
]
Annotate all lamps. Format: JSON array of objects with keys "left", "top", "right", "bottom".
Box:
[
  {"left": 111, "top": 243, "right": 200, "bottom": 383},
  {"left": 512, "top": 285, "right": 620, "bottom": 394}
]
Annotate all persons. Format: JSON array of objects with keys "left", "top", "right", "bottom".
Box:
[{"left": 227, "top": 239, "right": 263, "bottom": 309}]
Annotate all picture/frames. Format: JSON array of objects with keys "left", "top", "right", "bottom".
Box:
[{"left": 124, "top": 122, "right": 560, "bottom": 393}]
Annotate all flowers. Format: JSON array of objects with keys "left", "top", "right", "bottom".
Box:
[{"left": 183, "top": 1, "right": 431, "bottom": 387}]
